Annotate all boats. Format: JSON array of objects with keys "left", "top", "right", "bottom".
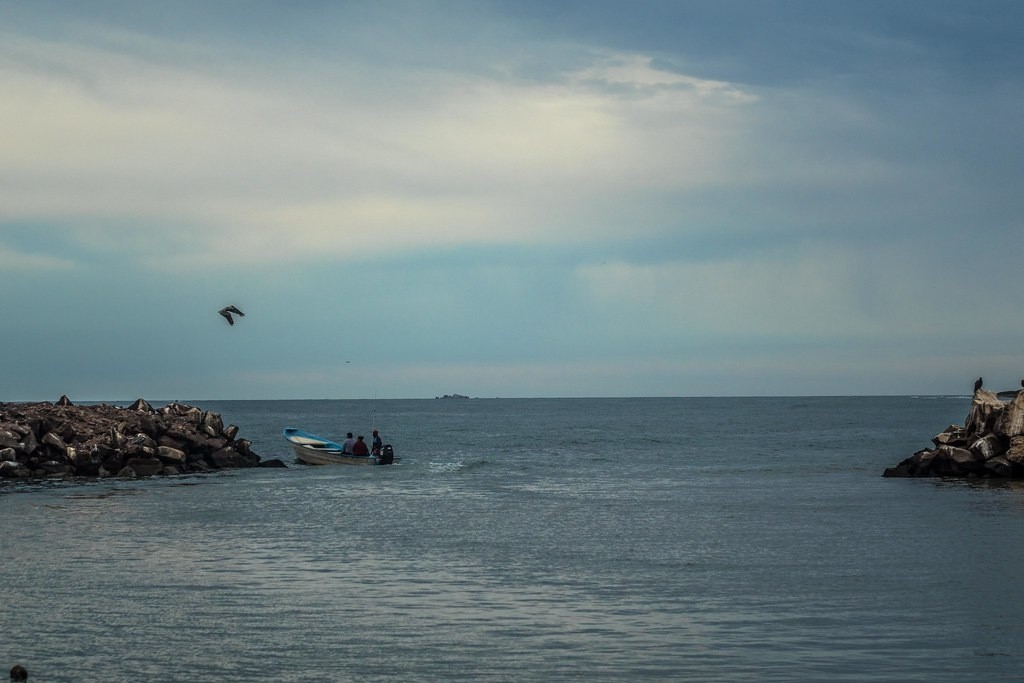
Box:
[{"left": 283, "top": 426, "right": 403, "bottom": 466}]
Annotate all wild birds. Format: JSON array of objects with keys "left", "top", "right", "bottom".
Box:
[
  {"left": 218, "top": 306, "right": 244, "bottom": 326},
  {"left": 974, "top": 377, "right": 983, "bottom": 392}
]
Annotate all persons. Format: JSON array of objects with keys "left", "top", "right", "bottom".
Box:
[
  {"left": 370, "top": 430, "right": 383, "bottom": 455},
  {"left": 352, "top": 435, "right": 370, "bottom": 456},
  {"left": 341, "top": 433, "right": 357, "bottom": 455}
]
